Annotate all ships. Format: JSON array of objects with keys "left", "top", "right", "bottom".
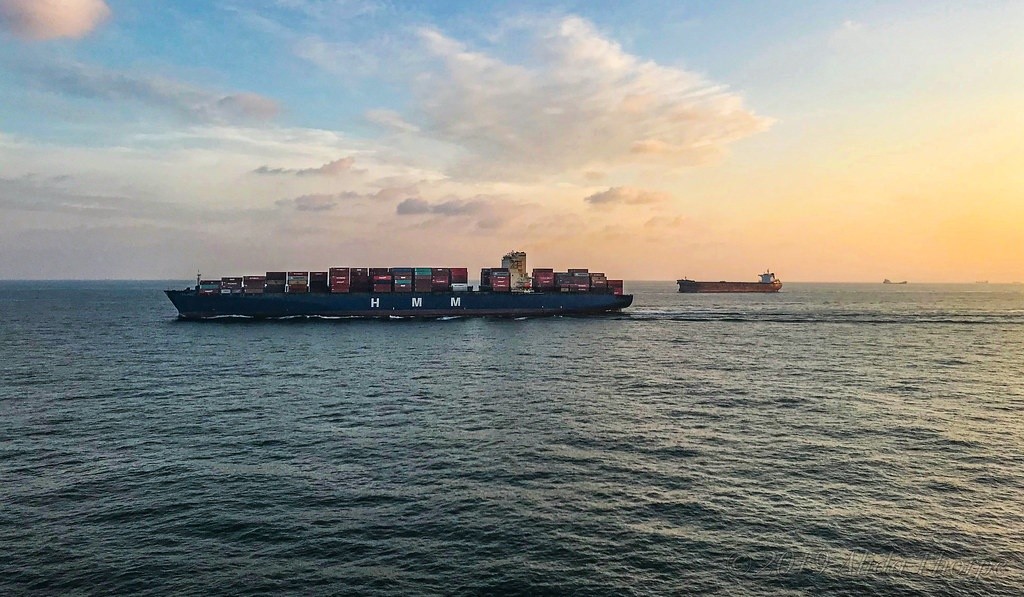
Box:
[
  {"left": 677, "top": 269, "right": 783, "bottom": 293},
  {"left": 162, "top": 249, "right": 635, "bottom": 322}
]
